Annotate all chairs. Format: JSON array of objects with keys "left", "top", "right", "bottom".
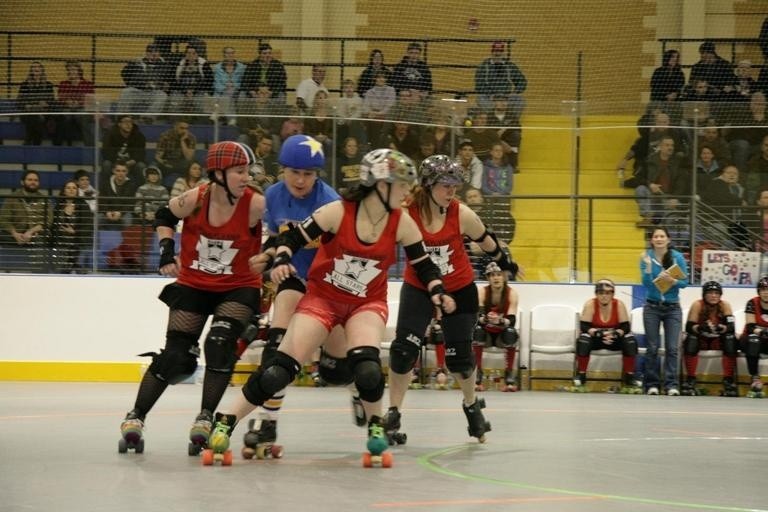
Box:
[
  {"left": 422, "top": 343, "right": 445, "bottom": 369},
  {"left": 524, "top": 307, "right": 580, "bottom": 389},
  {"left": 629, "top": 307, "right": 683, "bottom": 372},
  {"left": 380, "top": 300, "right": 418, "bottom": 387},
  {"left": 481, "top": 304, "right": 523, "bottom": 388}
]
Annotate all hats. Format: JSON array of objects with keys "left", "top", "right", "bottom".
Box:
[
  {"left": 141, "top": 164, "right": 163, "bottom": 182},
  {"left": 481, "top": 261, "right": 503, "bottom": 276},
  {"left": 459, "top": 139, "right": 472, "bottom": 145},
  {"left": 594, "top": 275, "right": 615, "bottom": 294},
  {"left": 492, "top": 42, "right": 505, "bottom": 53}
]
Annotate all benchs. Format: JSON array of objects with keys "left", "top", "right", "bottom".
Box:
[
  {"left": 2, "top": 171, "right": 188, "bottom": 201},
  {"left": 2, "top": 231, "right": 512, "bottom": 277},
  {"left": 3, "top": 101, "right": 115, "bottom": 119},
  {"left": 2, "top": 121, "right": 239, "bottom": 145},
  {"left": 2, "top": 145, "right": 211, "bottom": 168}
]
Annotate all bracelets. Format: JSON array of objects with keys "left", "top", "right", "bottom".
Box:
[{"left": 669, "top": 279, "right": 675, "bottom": 284}]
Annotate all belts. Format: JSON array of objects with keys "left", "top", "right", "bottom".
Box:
[{"left": 646, "top": 298, "right": 679, "bottom": 307}]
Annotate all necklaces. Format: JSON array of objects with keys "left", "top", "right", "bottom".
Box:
[{"left": 360, "top": 198, "right": 389, "bottom": 242}]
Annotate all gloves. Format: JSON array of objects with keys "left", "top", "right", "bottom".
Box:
[
  {"left": 157, "top": 237, "right": 178, "bottom": 269},
  {"left": 610, "top": 330, "right": 621, "bottom": 341},
  {"left": 760, "top": 328, "right": 768, "bottom": 339},
  {"left": 696, "top": 322, "right": 713, "bottom": 334},
  {"left": 261, "top": 253, "right": 275, "bottom": 273},
  {"left": 273, "top": 250, "right": 293, "bottom": 268},
  {"left": 496, "top": 252, "right": 519, "bottom": 278},
  {"left": 715, "top": 322, "right": 724, "bottom": 332},
  {"left": 429, "top": 285, "right": 453, "bottom": 300},
  {"left": 493, "top": 318, "right": 505, "bottom": 327},
  {"left": 594, "top": 330, "right": 605, "bottom": 340}
]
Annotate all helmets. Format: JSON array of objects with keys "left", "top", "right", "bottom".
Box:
[
  {"left": 277, "top": 134, "right": 326, "bottom": 172},
  {"left": 756, "top": 277, "right": 768, "bottom": 295},
  {"left": 417, "top": 153, "right": 464, "bottom": 216},
  {"left": 703, "top": 279, "right": 723, "bottom": 301},
  {"left": 356, "top": 147, "right": 417, "bottom": 214},
  {"left": 205, "top": 141, "right": 257, "bottom": 207}
]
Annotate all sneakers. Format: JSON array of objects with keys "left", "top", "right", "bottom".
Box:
[
  {"left": 666, "top": 384, "right": 681, "bottom": 397},
  {"left": 646, "top": 385, "right": 660, "bottom": 395}
]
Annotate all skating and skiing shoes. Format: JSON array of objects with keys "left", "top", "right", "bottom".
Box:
[
  {"left": 433, "top": 367, "right": 449, "bottom": 388},
  {"left": 185, "top": 410, "right": 213, "bottom": 459},
  {"left": 570, "top": 369, "right": 587, "bottom": 391},
  {"left": 198, "top": 409, "right": 238, "bottom": 471},
  {"left": 380, "top": 405, "right": 408, "bottom": 449},
  {"left": 308, "top": 361, "right": 330, "bottom": 389},
  {"left": 239, "top": 415, "right": 286, "bottom": 464},
  {"left": 749, "top": 373, "right": 763, "bottom": 395},
  {"left": 361, "top": 416, "right": 395, "bottom": 469},
  {"left": 678, "top": 376, "right": 699, "bottom": 396},
  {"left": 462, "top": 398, "right": 493, "bottom": 444},
  {"left": 617, "top": 373, "right": 633, "bottom": 392},
  {"left": 472, "top": 366, "right": 485, "bottom": 391},
  {"left": 115, "top": 407, "right": 148, "bottom": 458},
  {"left": 719, "top": 375, "right": 738, "bottom": 398},
  {"left": 406, "top": 369, "right": 420, "bottom": 387},
  {"left": 350, "top": 388, "right": 369, "bottom": 426},
  {"left": 501, "top": 369, "right": 519, "bottom": 393}
]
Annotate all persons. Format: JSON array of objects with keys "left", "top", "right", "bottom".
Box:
[
  {"left": 198, "top": 146, "right": 458, "bottom": 468},
  {"left": 725, "top": 91, "right": 765, "bottom": 169},
  {"left": 408, "top": 131, "right": 438, "bottom": 166},
  {"left": 57, "top": 59, "right": 96, "bottom": 145},
  {"left": 616, "top": 90, "right": 682, "bottom": 180},
  {"left": 73, "top": 167, "right": 98, "bottom": 210},
  {"left": 237, "top": 277, "right": 276, "bottom": 362},
  {"left": 488, "top": 92, "right": 522, "bottom": 175},
  {"left": 427, "top": 101, "right": 466, "bottom": 156},
  {"left": 115, "top": 44, "right": 171, "bottom": 122},
  {"left": 149, "top": 120, "right": 202, "bottom": 171},
  {"left": 687, "top": 41, "right": 735, "bottom": 90},
  {"left": 751, "top": 185, "right": 768, "bottom": 257},
  {"left": 303, "top": 88, "right": 335, "bottom": 148},
  {"left": 723, "top": 59, "right": 759, "bottom": 99},
  {"left": 238, "top": 82, "right": 280, "bottom": 154},
  {"left": 461, "top": 112, "right": 517, "bottom": 158},
  {"left": 356, "top": 47, "right": 395, "bottom": 92},
  {"left": 647, "top": 49, "right": 684, "bottom": 102},
  {"left": 2, "top": 169, "right": 55, "bottom": 274},
  {"left": 476, "top": 259, "right": 522, "bottom": 392},
  {"left": 689, "top": 122, "right": 733, "bottom": 170},
  {"left": 697, "top": 144, "right": 722, "bottom": 194},
  {"left": 50, "top": 180, "right": 94, "bottom": 276},
  {"left": 569, "top": 276, "right": 646, "bottom": 391},
  {"left": 381, "top": 150, "right": 526, "bottom": 447},
  {"left": 451, "top": 137, "right": 484, "bottom": 196},
  {"left": 641, "top": 226, "right": 690, "bottom": 394},
  {"left": 168, "top": 44, "right": 214, "bottom": 121},
  {"left": 740, "top": 276, "right": 768, "bottom": 395},
  {"left": 100, "top": 162, "right": 136, "bottom": 227},
  {"left": 680, "top": 279, "right": 742, "bottom": 396},
  {"left": 207, "top": 44, "right": 247, "bottom": 128},
  {"left": 276, "top": 107, "right": 307, "bottom": 143},
  {"left": 745, "top": 135, "right": 768, "bottom": 191},
  {"left": 615, "top": 113, "right": 685, "bottom": 189},
  {"left": 15, "top": 60, "right": 57, "bottom": 146},
  {"left": 635, "top": 136, "right": 684, "bottom": 225},
  {"left": 242, "top": 133, "right": 278, "bottom": 193},
  {"left": 702, "top": 166, "right": 750, "bottom": 240},
  {"left": 238, "top": 43, "right": 289, "bottom": 112},
  {"left": 296, "top": 62, "right": 331, "bottom": 112},
  {"left": 390, "top": 42, "right": 433, "bottom": 90},
  {"left": 241, "top": 133, "right": 368, "bottom": 463},
  {"left": 388, "top": 115, "right": 418, "bottom": 151},
  {"left": 472, "top": 40, "right": 527, "bottom": 123},
  {"left": 410, "top": 309, "right": 449, "bottom": 389},
  {"left": 136, "top": 164, "right": 170, "bottom": 224},
  {"left": 170, "top": 160, "right": 209, "bottom": 202},
  {"left": 101, "top": 115, "right": 145, "bottom": 176},
  {"left": 462, "top": 185, "right": 490, "bottom": 217},
  {"left": 363, "top": 68, "right": 397, "bottom": 135},
  {"left": 377, "top": 88, "right": 430, "bottom": 134},
  {"left": 336, "top": 134, "right": 366, "bottom": 196},
  {"left": 116, "top": 139, "right": 264, "bottom": 454},
  {"left": 483, "top": 142, "right": 514, "bottom": 195},
  {"left": 332, "top": 78, "right": 362, "bottom": 131},
  {"left": 682, "top": 77, "right": 720, "bottom": 105}
]
